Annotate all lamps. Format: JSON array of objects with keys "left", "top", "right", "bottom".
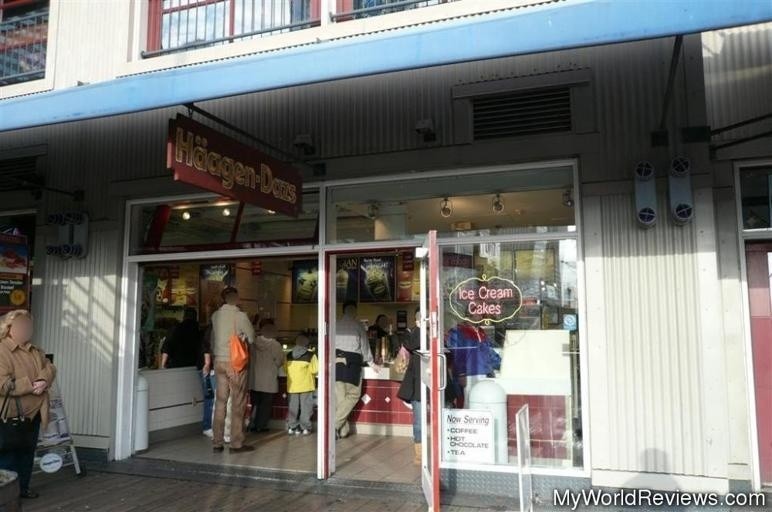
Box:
[{"left": 364, "top": 183, "right": 576, "bottom": 222}]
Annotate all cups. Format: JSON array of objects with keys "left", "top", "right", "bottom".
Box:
[
  {"left": 296, "top": 274, "right": 318, "bottom": 303},
  {"left": 336, "top": 274, "right": 349, "bottom": 301},
  {"left": 365, "top": 272, "right": 392, "bottom": 302},
  {"left": 398, "top": 267, "right": 412, "bottom": 299}
]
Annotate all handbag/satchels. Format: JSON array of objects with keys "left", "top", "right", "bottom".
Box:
[
  {"left": 228, "top": 334, "right": 250, "bottom": 376},
  {"left": 0, "top": 412, "right": 33, "bottom": 454}
]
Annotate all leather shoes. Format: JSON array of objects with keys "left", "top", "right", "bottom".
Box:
[
  {"left": 229, "top": 444, "right": 256, "bottom": 454},
  {"left": 21, "top": 489, "right": 39, "bottom": 500},
  {"left": 212, "top": 446, "right": 224, "bottom": 454}
]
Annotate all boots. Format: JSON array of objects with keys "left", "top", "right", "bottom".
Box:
[{"left": 414, "top": 442, "right": 422, "bottom": 464}]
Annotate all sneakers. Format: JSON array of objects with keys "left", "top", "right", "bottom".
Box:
[
  {"left": 202, "top": 428, "right": 214, "bottom": 441},
  {"left": 302, "top": 428, "right": 312, "bottom": 435},
  {"left": 287, "top": 426, "right": 301, "bottom": 434}
]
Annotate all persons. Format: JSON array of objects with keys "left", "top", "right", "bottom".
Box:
[
  {"left": 397, "top": 308, "right": 462, "bottom": 465},
  {"left": 160, "top": 286, "right": 286, "bottom": 454},
  {"left": 335, "top": 300, "right": 382, "bottom": 440},
  {"left": 446, "top": 304, "right": 502, "bottom": 406},
  {"left": 283, "top": 334, "right": 319, "bottom": 435},
  {"left": 0, "top": 309, "right": 58, "bottom": 499},
  {"left": 369, "top": 314, "right": 391, "bottom": 346}
]
